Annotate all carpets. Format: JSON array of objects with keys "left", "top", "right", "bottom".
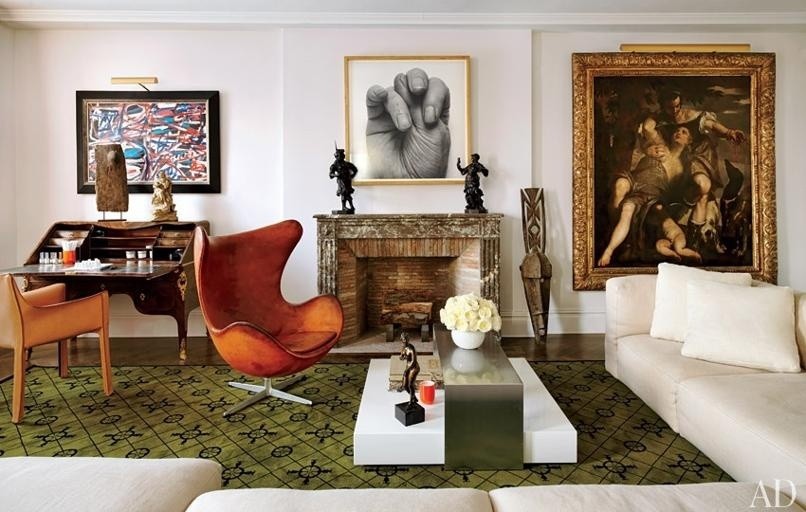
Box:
[{"left": 0, "top": 359, "right": 735, "bottom": 492}]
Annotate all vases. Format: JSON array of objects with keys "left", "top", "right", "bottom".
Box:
[
  {"left": 450, "top": 347, "right": 486, "bottom": 373},
  {"left": 451, "top": 326, "right": 487, "bottom": 350}
]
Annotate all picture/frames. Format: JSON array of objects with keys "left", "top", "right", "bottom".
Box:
[
  {"left": 76, "top": 90, "right": 221, "bottom": 194},
  {"left": 571, "top": 52, "right": 777, "bottom": 290},
  {"left": 344, "top": 55, "right": 474, "bottom": 187}
]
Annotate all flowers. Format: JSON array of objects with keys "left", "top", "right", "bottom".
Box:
[{"left": 439, "top": 293, "right": 502, "bottom": 334}]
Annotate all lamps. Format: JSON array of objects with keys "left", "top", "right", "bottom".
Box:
[{"left": 112, "top": 76, "right": 158, "bottom": 91}]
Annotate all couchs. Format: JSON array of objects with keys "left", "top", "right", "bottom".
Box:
[
  {"left": 0, "top": 456, "right": 806, "bottom": 512},
  {"left": 603, "top": 269, "right": 806, "bottom": 512}
]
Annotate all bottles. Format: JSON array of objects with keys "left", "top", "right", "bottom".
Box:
[
  {"left": 125, "top": 261, "right": 154, "bottom": 272},
  {"left": 125, "top": 250, "right": 153, "bottom": 260},
  {"left": 38, "top": 251, "right": 64, "bottom": 264}
]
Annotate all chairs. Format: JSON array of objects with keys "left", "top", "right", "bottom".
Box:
[
  {"left": 0, "top": 274, "right": 114, "bottom": 425},
  {"left": 193, "top": 219, "right": 345, "bottom": 418}
]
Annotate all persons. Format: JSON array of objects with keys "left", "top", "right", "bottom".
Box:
[
  {"left": 364, "top": 68, "right": 451, "bottom": 178},
  {"left": 399, "top": 333, "right": 421, "bottom": 410},
  {"left": 328, "top": 149, "right": 359, "bottom": 211},
  {"left": 597, "top": 84, "right": 752, "bottom": 266},
  {"left": 455, "top": 153, "right": 489, "bottom": 213}
]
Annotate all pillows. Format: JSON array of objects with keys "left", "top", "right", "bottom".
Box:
[
  {"left": 681, "top": 278, "right": 802, "bottom": 374},
  {"left": 650, "top": 262, "right": 752, "bottom": 343}
]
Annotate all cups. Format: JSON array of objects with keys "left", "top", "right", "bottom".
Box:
[
  {"left": 418, "top": 380, "right": 437, "bottom": 405},
  {"left": 62, "top": 241, "right": 78, "bottom": 264}
]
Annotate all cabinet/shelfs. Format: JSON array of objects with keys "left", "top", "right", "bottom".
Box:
[{"left": 20, "top": 220, "right": 212, "bottom": 361}]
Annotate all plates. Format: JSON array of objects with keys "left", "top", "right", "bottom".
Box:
[{"left": 62, "top": 263, "right": 113, "bottom": 272}]
